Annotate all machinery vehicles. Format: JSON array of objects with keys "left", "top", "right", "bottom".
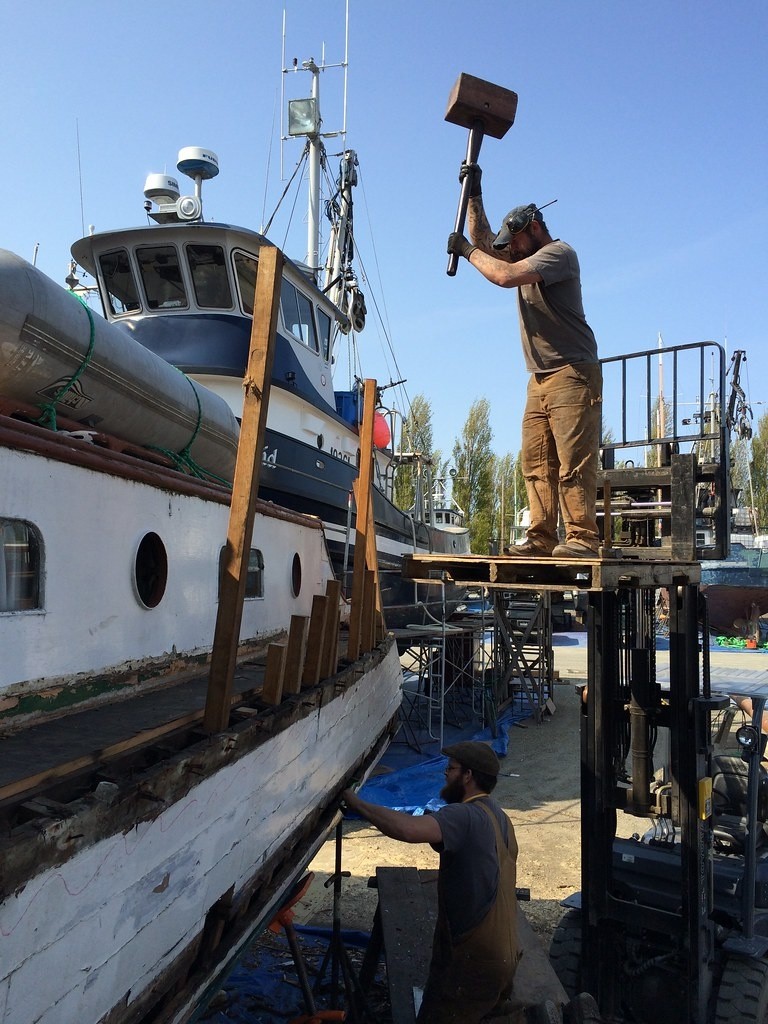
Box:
[{"left": 547, "top": 339, "right": 768, "bottom": 1024}]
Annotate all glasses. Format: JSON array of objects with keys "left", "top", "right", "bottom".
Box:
[{"left": 446, "top": 766, "right": 462, "bottom": 772}]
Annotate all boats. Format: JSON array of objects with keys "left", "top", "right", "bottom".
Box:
[
  {"left": 0, "top": 1, "right": 473, "bottom": 1023},
  {"left": 511, "top": 330, "right": 767, "bottom": 635}
]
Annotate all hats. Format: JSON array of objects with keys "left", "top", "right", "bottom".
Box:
[
  {"left": 492, "top": 205, "right": 543, "bottom": 250},
  {"left": 441, "top": 742, "right": 500, "bottom": 777}
]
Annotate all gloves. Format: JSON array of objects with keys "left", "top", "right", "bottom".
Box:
[
  {"left": 459, "top": 159, "right": 482, "bottom": 198},
  {"left": 447, "top": 232, "right": 477, "bottom": 262}
]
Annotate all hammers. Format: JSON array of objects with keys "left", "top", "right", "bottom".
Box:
[{"left": 443, "top": 70, "right": 519, "bottom": 279}]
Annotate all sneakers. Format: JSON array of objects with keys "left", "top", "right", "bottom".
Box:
[
  {"left": 509, "top": 540, "right": 552, "bottom": 557},
  {"left": 552, "top": 542, "right": 599, "bottom": 558}
]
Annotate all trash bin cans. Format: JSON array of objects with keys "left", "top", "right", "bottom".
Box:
[{"left": 334, "top": 391, "right": 363, "bottom": 427}]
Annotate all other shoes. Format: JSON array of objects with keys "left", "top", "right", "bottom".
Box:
[
  {"left": 526, "top": 999, "right": 561, "bottom": 1024},
  {"left": 562, "top": 992, "right": 602, "bottom": 1024}
]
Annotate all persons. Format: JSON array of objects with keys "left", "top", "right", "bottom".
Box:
[
  {"left": 711, "top": 694, "right": 768, "bottom": 817},
  {"left": 336, "top": 741, "right": 601, "bottom": 1024},
  {"left": 447, "top": 160, "right": 603, "bottom": 557}
]
